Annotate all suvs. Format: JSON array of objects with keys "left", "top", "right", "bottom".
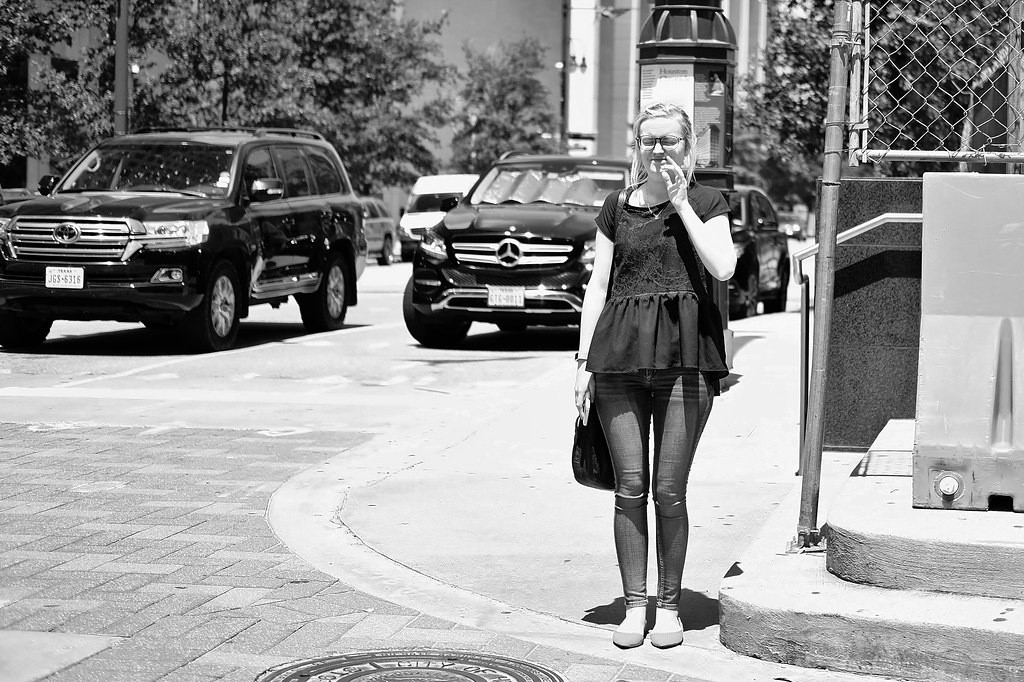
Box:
[
  {"left": 398, "top": 174, "right": 481, "bottom": 262},
  {"left": 0, "top": 124, "right": 367, "bottom": 352},
  {"left": 402, "top": 150, "right": 791, "bottom": 347}
]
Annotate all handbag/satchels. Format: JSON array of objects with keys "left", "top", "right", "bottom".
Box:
[{"left": 570, "top": 384, "right": 651, "bottom": 494}]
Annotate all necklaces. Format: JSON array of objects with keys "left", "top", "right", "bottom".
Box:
[{"left": 645, "top": 182, "right": 670, "bottom": 219}]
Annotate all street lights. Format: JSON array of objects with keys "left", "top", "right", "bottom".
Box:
[{"left": 558, "top": 55, "right": 587, "bottom": 143}]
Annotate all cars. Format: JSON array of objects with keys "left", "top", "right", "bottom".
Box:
[{"left": 358, "top": 196, "right": 396, "bottom": 265}]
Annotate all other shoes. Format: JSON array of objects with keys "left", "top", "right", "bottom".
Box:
[
  {"left": 650, "top": 609, "right": 684, "bottom": 649},
  {"left": 612, "top": 615, "right": 648, "bottom": 648}
]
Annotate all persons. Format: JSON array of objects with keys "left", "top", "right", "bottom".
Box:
[
  {"left": 712, "top": 74, "right": 724, "bottom": 93},
  {"left": 574, "top": 103, "right": 737, "bottom": 648}
]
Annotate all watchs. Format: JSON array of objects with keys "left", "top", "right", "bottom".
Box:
[{"left": 575, "top": 352, "right": 588, "bottom": 362}]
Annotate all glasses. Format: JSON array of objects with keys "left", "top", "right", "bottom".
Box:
[{"left": 636, "top": 134, "right": 686, "bottom": 152}]
